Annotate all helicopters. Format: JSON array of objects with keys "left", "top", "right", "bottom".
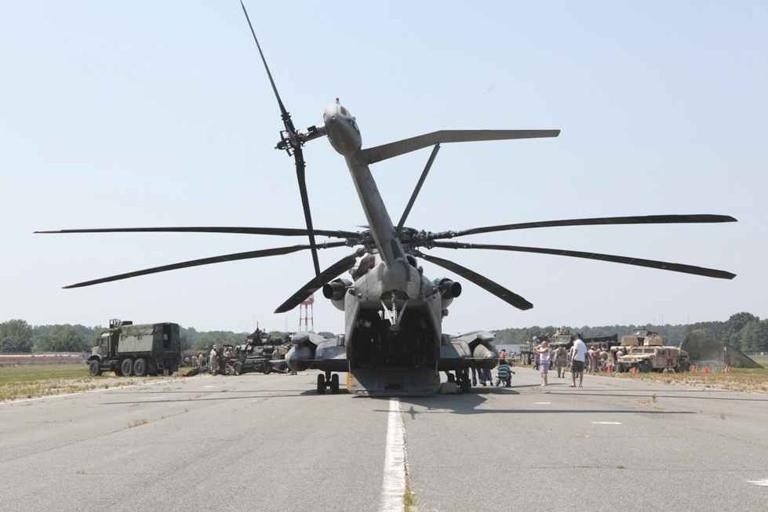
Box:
[{"left": 29, "top": 0, "right": 737, "bottom": 399}]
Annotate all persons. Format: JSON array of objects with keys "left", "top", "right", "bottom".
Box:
[
  {"left": 183, "top": 345, "right": 246, "bottom": 376},
  {"left": 272, "top": 344, "right": 298, "bottom": 375},
  {"left": 445, "top": 333, "right": 627, "bottom": 388}
]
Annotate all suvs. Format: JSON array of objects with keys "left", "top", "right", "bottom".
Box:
[{"left": 607, "top": 330, "right": 694, "bottom": 373}]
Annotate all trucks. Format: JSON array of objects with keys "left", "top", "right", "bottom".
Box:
[
  {"left": 548, "top": 330, "right": 618, "bottom": 356},
  {"left": 84, "top": 316, "right": 183, "bottom": 380}
]
